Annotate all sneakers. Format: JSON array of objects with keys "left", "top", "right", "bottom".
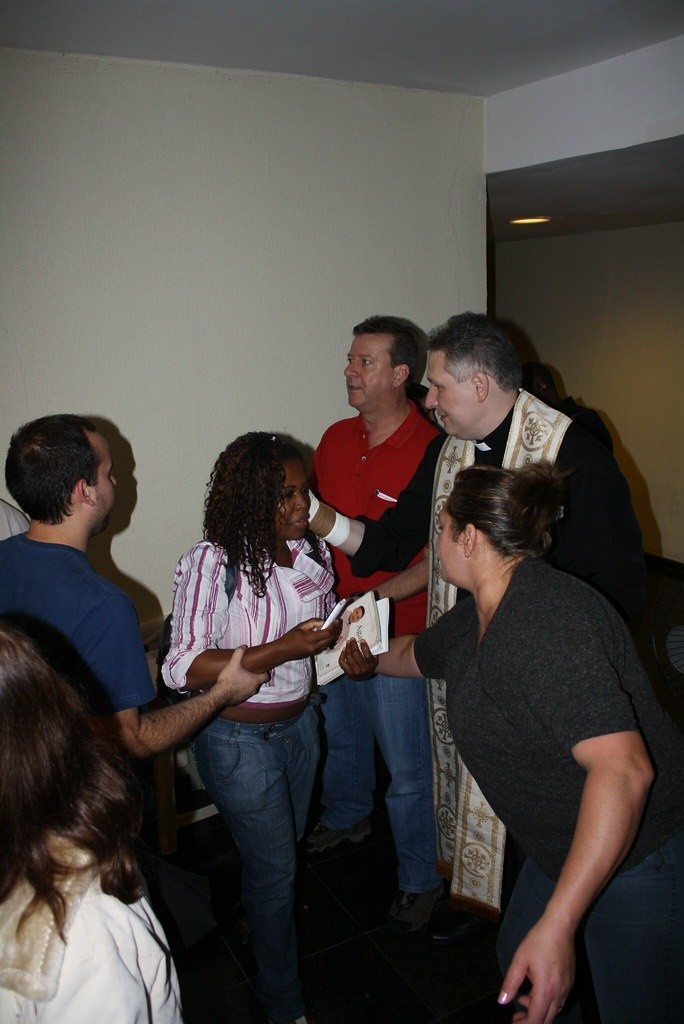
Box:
[
  {"left": 385, "top": 881, "right": 444, "bottom": 932},
  {"left": 303, "top": 816, "right": 371, "bottom": 851}
]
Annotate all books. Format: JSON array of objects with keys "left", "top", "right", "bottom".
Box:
[{"left": 313, "top": 589, "right": 390, "bottom": 687}]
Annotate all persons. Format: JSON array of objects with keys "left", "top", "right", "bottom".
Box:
[
  {"left": 307, "top": 315, "right": 445, "bottom": 938},
  {"left": 327, "top": 605, "right": 365, "bottom": 650},
  {"left": 307, "top": 311, "right": 647, "bottom": 949},
  {"left": 0, "top": 416, "right": 272, "bottom": 763},
  {"left": 0, "top": 618, "right": 185, "bottom": 1024},
  {"left": 376, "top": 464, "right": 683, "bottom": 1024},
  {"left": 159, "top": 430, "right": 379, "bottom": 1024}
]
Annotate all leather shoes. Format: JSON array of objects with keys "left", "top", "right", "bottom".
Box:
[{"left": 432, "top": 914, "right": 503, "bottom": 943}]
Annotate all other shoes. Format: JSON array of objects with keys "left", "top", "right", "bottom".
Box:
[
  {"left": 239, "top": 914, "right": 252, "bottom": 944},
  {"left": 267, "top": 1013, "right": 319, "bottom": 1024}
]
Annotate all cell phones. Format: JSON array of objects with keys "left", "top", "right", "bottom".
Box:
[{"left": 321, "top": 599, "right": 347, "bottom": 630}]
